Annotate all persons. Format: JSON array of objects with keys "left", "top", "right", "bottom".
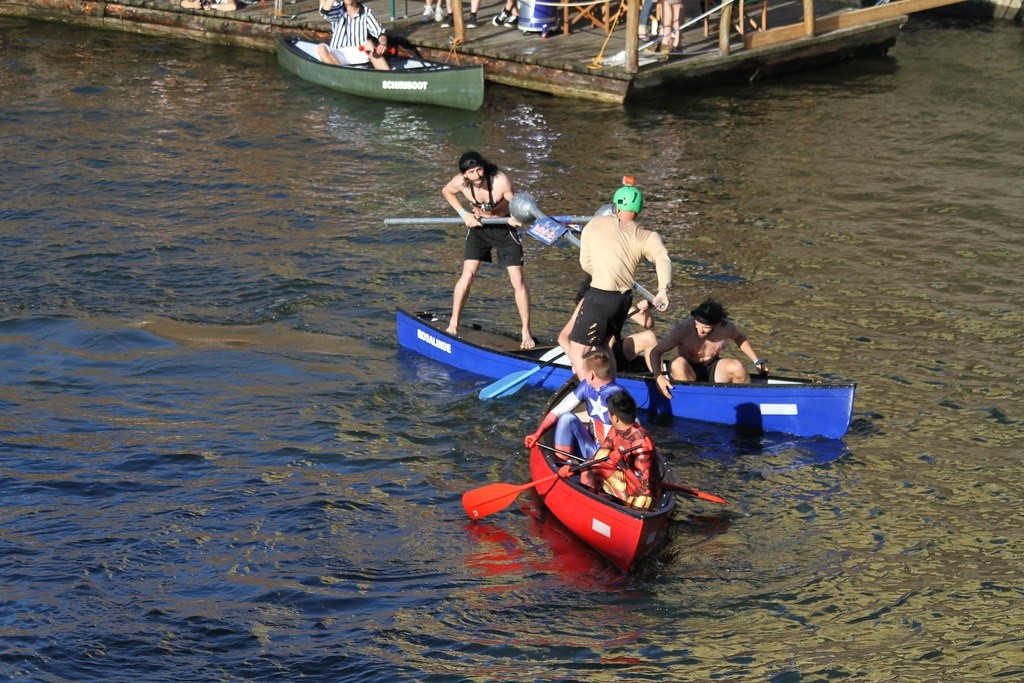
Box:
[
  {"left": 422, "top": 0, "right": 444, "bottom": 22},
  {"left": 441, "top": 0, "right": 480, "bottom": 30},
  {"left": 639, "top": 0, "right": 660, "bottom": 42},
  {"left": 181, "top": 0, "right": 237, "bottom": 12},
  {"left": 442, "top": 151, "right": 537, "bottom": 350},
  {"left": 559, "top": 391, "right": 662, "bottom": 511},
  {"left": 317, "top": 0, "right": 392, "bottom": 71},
  {"left": 525, "top": 350, "right": 639, "bottom": 461},
  {"left": 492, "top": 0, "right": 520, "bottom": 27},
  {"left": 567, "top": 185, "right": 672, "bottom": 385},
  {"left": 557, "top": 274, "right": 668, "bottom": 382},
  {"left": 649, "top": 302, "right": 769, "bottom": 400},
  {"left": 662, "top": 0, "right": 683, "bottom": 48}
]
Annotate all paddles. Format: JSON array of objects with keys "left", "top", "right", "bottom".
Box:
[
  {"left": 461, "top": 436, "right": 654, "bottom": 521},
  {"left": 526, "top": 438, "right": 727, "bottom": 505},
  {"left": 756, "top": 358, "right": 769, "bottom": 376},
  {"left": 478, "top": 301, "right": 653, "bottom": 401}
]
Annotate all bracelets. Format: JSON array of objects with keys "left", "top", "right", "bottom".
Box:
[
  {"left": 459, "top": 209, "right": 465, "bottom": 217},
  {"left": 754, "top": 360, "right": 760, "bottom": 366}
]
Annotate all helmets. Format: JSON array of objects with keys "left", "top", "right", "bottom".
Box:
[{"left": 612, "top": 185, "right": 643, "bottom": 214}]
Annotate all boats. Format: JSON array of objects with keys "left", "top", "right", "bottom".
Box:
[
  {"left": 530, "top": 372, "right": 676, "bottom": 574},
  {"left": 397, "top": 305, "right": 857, "bottom": 442},
  {"left": 274, "top": 33, "right": 485, "bottom": 110}
]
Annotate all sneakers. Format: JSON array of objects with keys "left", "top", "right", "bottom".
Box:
[
  {"left": 644, "top": 44, "right": 682, "bottom": 55},
  {"left": 441, "top": 15, "right": 454, "bottom": 28},
  {"left": 467, "top": 15, "right": 478, "bottom": 28},
  {"left": 492, "top": 7, "right": 511, "bottom": 26},
  {"left": 434, "top": 8, "right": 444, "bottom": 22},
  {"left": 504, "top": 16, "right": 518, "bottom": 28},
  {"left": 420, "top": 6, "right": 434, "bottom": 22}
]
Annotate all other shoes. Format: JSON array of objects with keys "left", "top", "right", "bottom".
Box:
[{"left": 639, "top": 34, "right": 650, "bottom": 44}]
